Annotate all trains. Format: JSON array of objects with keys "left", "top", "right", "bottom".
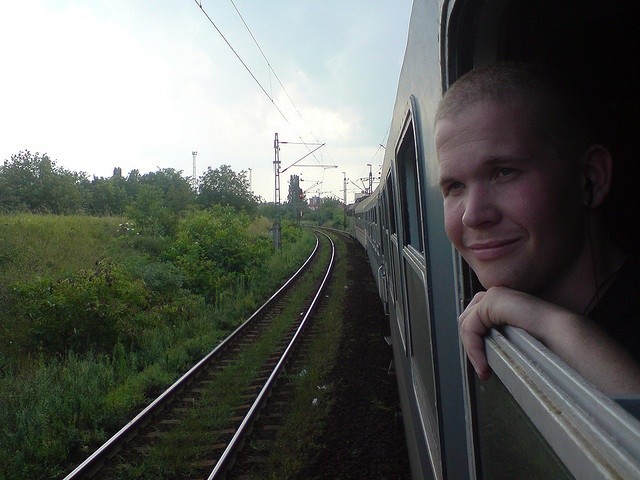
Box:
[{"left": 346, "top": 1, "right": 640, "bottom": 479}]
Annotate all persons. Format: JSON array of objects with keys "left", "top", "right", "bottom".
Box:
[{"left": 435, "top": 59, "right": 640, "bottom": 400}]
